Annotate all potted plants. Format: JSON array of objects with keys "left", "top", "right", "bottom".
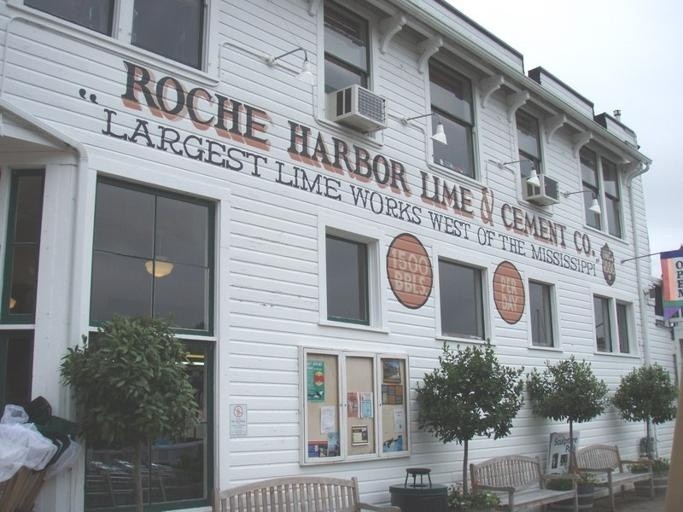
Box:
[
  {"left": 547, "top": 470, "right": 601, "bottom": 512},
  {"left": 627, "top": 456, "right": 672, "bottom": 498}
]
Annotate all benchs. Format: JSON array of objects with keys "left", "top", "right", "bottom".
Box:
[
  {"left": 470, "top": 454, "right": 579, "bottom": 511},
  {"left": 215, "top": 475, "right": 400, "bottom": 512},
  {"left": 569, "top": 444, "right": 655, "bottom": 512}
]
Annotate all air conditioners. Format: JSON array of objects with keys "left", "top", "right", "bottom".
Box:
[
  {"left": 519, "top": 173, "right": 560, "bottom": 207},
  {"left": 328, "top": 83, "right": 388, "bottom": 134}
]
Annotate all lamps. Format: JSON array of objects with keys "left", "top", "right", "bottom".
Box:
[
  {"left": 401, "top": 112, "right": 447, "bottom": 146},
  {"left": 563, "top": 190, "right": 602, "bottom": 215},
  {"left": 264, "top": 45, "right": 316, "bottom": 88},
  {"left": 144, "top": 228, "right": 173, "bottom": 278},
  {"left": 498, "top": 158, "right": 541, "bottom": 188}
]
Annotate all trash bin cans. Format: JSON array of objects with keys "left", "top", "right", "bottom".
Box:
[{"left": 390, "top": 468, "right": 449, "bottom": 512}]
